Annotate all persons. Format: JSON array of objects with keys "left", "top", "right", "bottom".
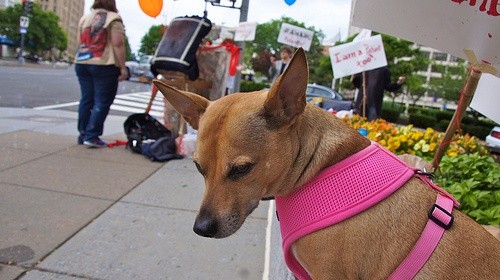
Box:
[
  {"left": 349, "top": 67, "right": 406, "bottom": 121},
  {"left": 268, "top": 47, "right": 292, "bottom": 83},
  {"left": 73, "top": 0, "right": 129, "bottom": 148}
]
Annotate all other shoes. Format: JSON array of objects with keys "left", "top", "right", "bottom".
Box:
[{"left": 84, "top": 138, "right": 107, "bottom": 148}]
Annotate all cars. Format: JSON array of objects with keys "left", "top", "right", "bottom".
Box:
[
  {"left": 305, "top": 82, "right": 355, "bottom": 115},
  {"left": 123, "top": 54, "right": 155, "bottom": 80}
]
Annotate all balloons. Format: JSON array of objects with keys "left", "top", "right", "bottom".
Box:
[
  {"left": 138, "top": 0, "right": 163, "bottom": 18},
  {"left": 285, "top": 0, "right": 296, "bottom": 5}
]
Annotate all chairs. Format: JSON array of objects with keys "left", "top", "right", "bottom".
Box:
[{"left": 144, "top": 77, "right": 214, "bottom": 159}]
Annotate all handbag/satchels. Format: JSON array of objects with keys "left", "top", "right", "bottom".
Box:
[{"left": 123, "top": 113, "right": 177, "bottom": 161}]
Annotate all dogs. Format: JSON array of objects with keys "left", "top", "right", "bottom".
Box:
[{"left": 153, "top": 48, "right": 500, "bottom": 280}]
